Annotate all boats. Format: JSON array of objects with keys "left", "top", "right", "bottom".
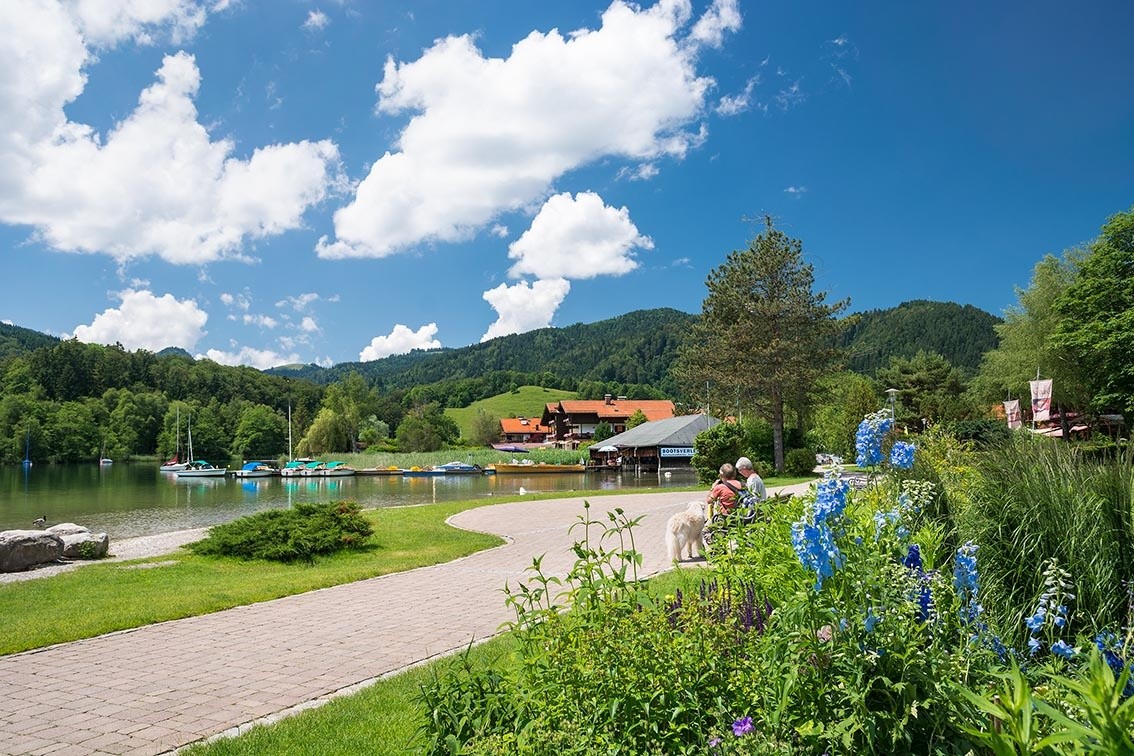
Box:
[
  {"left": 235, "top": 461, "right": 272, "bottom": 477},
  {"left": 355, "top": 466, "right": 405, "bottom": 475},
  {"left": 494, "top": 462, "right": 587, "bottom": 474},
  {"left": 432, "top": 461, "right": 483, "bottom": 474},
  {"left": 402, "top": 465, "right": 448, "bottom": 476}
]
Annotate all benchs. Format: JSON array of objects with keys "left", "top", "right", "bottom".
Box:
[
  {"left": 706, "top": 493, "right": 794, "bottom": 555},
  {"left": 848, "top": 471, "right": 878, "bottom": 498}
]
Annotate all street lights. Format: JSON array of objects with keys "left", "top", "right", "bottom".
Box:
[{"left": 885, "top": 388, "right": 899, "bottom": 432}]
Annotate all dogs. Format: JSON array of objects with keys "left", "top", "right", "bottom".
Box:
[{"left": 665, "top": 502, "right": 707, "bottom": 561}]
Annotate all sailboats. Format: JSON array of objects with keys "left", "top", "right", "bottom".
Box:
[
  {"left": 159, "top": 406, "right": 190, "bottom": 471},
  {"left": 22, "top": 425, "right": 32, "bottom": 463},
  {"left": 99, "top": 444, "right": 112, "bottom": 463},
  {"left": 176, "top": 413, "right": 227, "bottom": 476},
  {"left": 280, "top": 404, "right": 355, "bottom": 477}
]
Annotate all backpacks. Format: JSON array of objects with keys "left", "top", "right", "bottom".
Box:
[{"left": 723, "top": 481, "right": 760, "bottom": 524}]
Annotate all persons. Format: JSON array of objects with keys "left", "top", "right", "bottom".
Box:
[
  {"left": 606, "top": 455, "right": 634, "bottom": 466},
  {"left": 704, "top": 456, "right": 766, "bottom": 556}
]
